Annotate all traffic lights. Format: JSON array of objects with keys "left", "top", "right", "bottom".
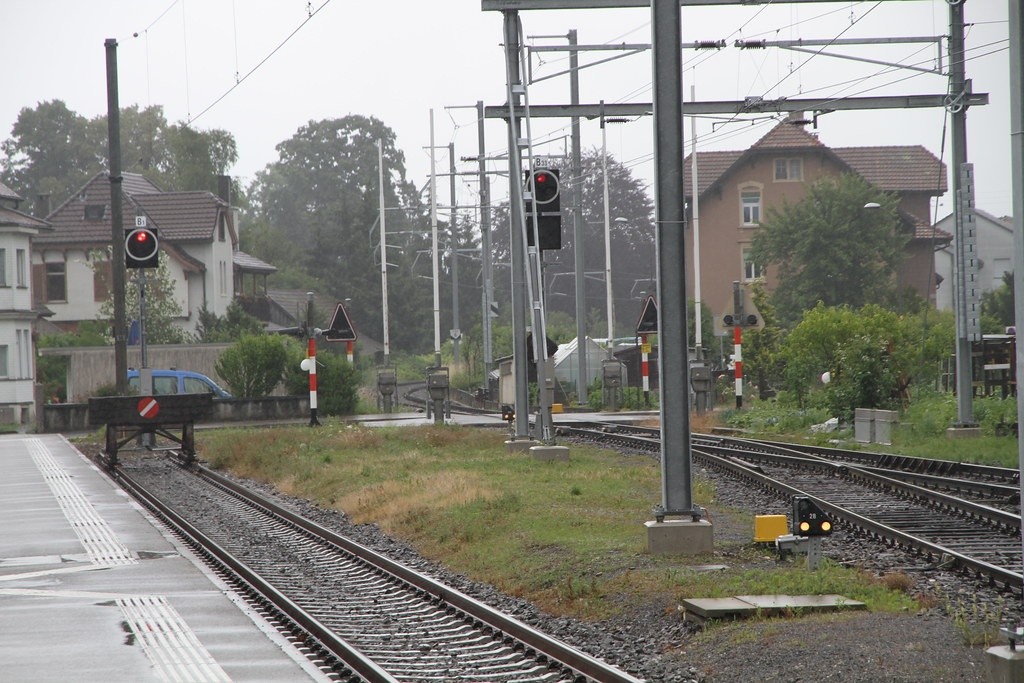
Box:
[
  {"left": 124, "top": 227, "right": 159, "bottom": 269},
  {"left": 523, "top": 168, "right": 562, "bottom": 250}
]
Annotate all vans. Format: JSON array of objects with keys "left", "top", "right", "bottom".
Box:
[{"left": 128, "top": 366, "right": 234, "bottom": 400}]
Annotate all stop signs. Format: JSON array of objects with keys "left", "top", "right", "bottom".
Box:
[{"left": 137, "top": 396, "right": 159, "bottom": 419}]
